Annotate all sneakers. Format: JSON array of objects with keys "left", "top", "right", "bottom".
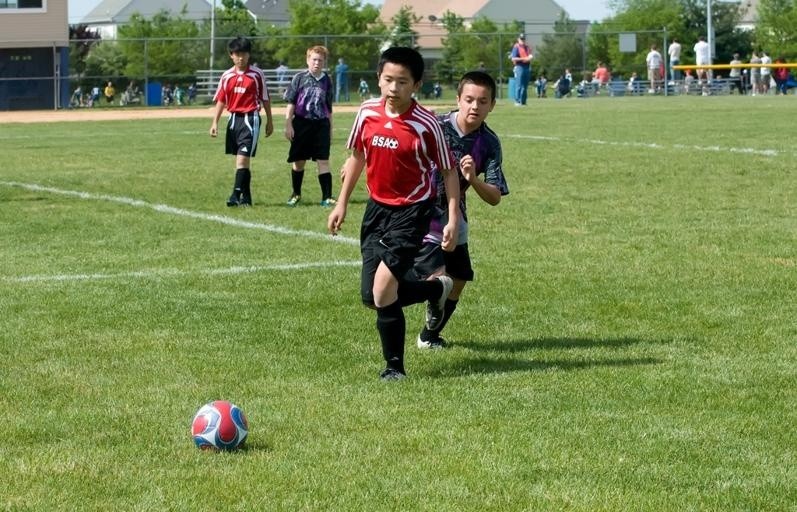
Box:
[
  {"left": 287, "top": 194, "right": 302, "bottom": 206},
  {"left": 418, "top": 333, "right": 447, "bottom": 350},
  {"left": 322, "top": 198, "right": 338, "bottom": 207},
  {"left": 380, "top": 368, "right": 406, "bottom": 382},
  {"left": 226, "top": 187, "right": 252, "bottom": 208},
  {"left": 424, "top": 275, "right": 453, "bottom": 331}
]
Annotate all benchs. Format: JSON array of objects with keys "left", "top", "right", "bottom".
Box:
[
  {"left": 606, "top": 78, "right": 740, "bottom": 97},
  {"left": 195, "top": 68, "right": 330, "bottom": 98}
]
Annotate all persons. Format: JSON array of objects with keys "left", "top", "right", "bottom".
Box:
[
  {"left": 285, "top": 45, "right": 336, "bottom": 206},
  {"left": 68, "top": 80, "right": 143, "bottom": 109},
  {"left": 358, "top": 77, "right": 370, "bottom": 104},
  {"left": 277, "top": 61, "right": 289, "bottom": 98},
  {"left": 533, "top": 34, "right": 790, "bottom": 98},
  {"left": 478, "top": 62, "right": 487, "bottom": 72},
  {"left": 433, "top": 81, "right": 442, "bottom": 100},
  {"left": 511, "top": 32, "right": 533, "bottom": 107},
  {"left": 161, "top": 80, "right": 198, "bottom": 106},
  {"left": 328, "top": 46, "right": 460, "bottom": 383},
  {"left": 209, "top": 36, "right": 273, "bottom": 206},
  {"left": 334, "top": 58, "right": 350, "bottom": 102},
  {"left": 406, "top": 70, "right": 511, "bottom": 351}
]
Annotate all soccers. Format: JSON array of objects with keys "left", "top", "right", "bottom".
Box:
[{"left": 192, "top": 400, "right": 248, "bottom": 450}]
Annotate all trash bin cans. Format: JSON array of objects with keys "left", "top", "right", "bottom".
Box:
[
  {"left": 144, "top": 82, "right": 162, "bottom": 107},
  {"left": 508, "top": 77, "right": 516, "bottom": 100}
]
